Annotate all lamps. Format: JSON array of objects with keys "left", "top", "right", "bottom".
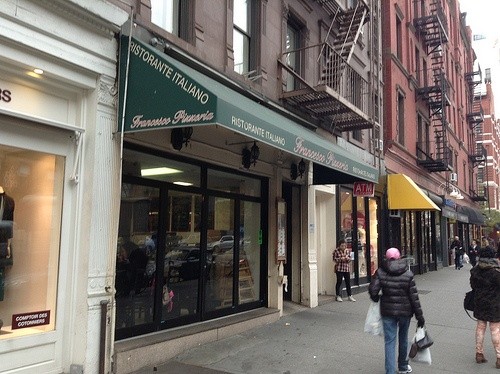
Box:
[
  {"left": 170, "top": 127, "right": 193, "bottom": 151},
  {"left": 241, "top": 140, "right": 259, "bottom": 168},
  {"left": 438, "top": 182, "right": 464, "bottom": 200},
  {"left": 289, "top": 158, "right": 305, "bottom": 180}
]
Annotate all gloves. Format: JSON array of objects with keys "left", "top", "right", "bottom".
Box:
[
  {"left": 370, "top": 296, "right": 379, "bottom": 302},
  {"left": 417, "top": 319, "right": 425, "bottom": 327}
]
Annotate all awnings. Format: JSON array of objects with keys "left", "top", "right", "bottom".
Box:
[
  {"left": 387, "top": 173, "right": 441, "bottom": 212},
  {"left": 461, "top": 206, "right": 484, "bottom": 225},
  {"left": 117, "top": 32, "right": 379, "bottom": 187}
]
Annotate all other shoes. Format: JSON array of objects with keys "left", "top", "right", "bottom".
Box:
[
  {"left": 337, "top": 295, "right": 342, "bottom": 301},
  {"left": 476, "top": 353, "right": 487, "bottom": 363},
  {"left": 496, "top": 358, "right": 500, "bottom": 368},
  {"left": 348, "top": 295, "right": 356, "bottom": 302}
]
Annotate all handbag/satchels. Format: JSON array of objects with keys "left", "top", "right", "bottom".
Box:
[
  {"left": 407, "top": 328, "right": 431, "bottom": 366},
  {"left": 363, "top": 300, "right": 383, "bottom": 335},
  {"left": 463, "top": 291, "right": 474, "bottom": 311},
  {"left": 415, "top": 326, "right": 433, "bottom": 350},
  {"left": 334, "top": 264, "right": 337, "bottom": 273}
]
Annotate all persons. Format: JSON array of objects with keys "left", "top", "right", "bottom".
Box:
[
  {"left": 450, "top": 236, "right": 495, "bottom": 270},
  {"left": 333, "top": 239, "right": 356, "bottom": 302},
  {"left": 368, "top": 248, "right": 425, "bottom": 374},
  {"left": 116, "top": 243, "right": 148, "bottom": 327},
  {"left": 469, "top": 246, "right": 500, "bottom": 368}
]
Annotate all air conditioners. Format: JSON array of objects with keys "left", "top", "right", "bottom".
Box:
[
  {"left": 451, "top": 173, "right": 458, "bottom": 182},
  {"left": 434, "top": 46, "right": 443, "bottom": 57}
]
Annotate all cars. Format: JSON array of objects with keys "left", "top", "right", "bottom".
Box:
[
  {"left": 211, "top": 234, "right": 243, "bottom": 254},
  {"left": 162, "top": 246, "right": 211, "bottom": 283}
]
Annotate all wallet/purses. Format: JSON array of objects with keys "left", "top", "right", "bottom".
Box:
[{"left": 409, "top": 337, "right": 425, "bottom": 358}]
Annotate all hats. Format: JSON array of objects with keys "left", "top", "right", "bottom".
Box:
[{"left": 385, "top": 247, "right": 400, "bottom": 260}]
